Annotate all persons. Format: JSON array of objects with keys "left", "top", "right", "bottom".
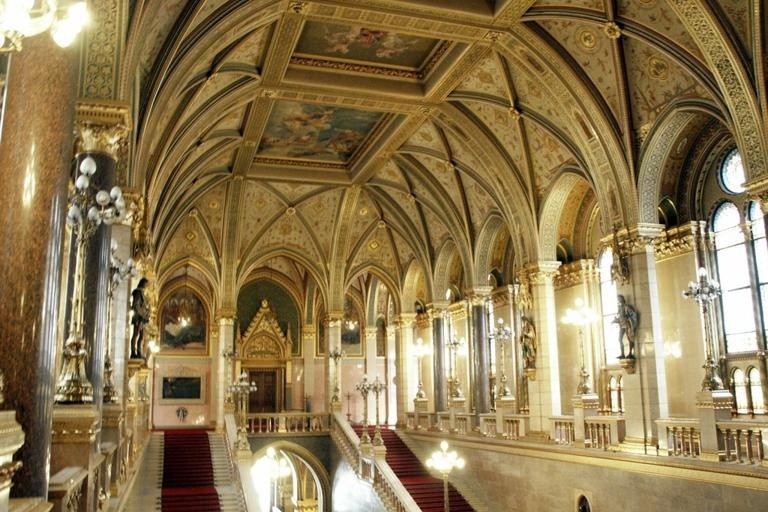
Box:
[
  {"left": 519, "top": 316, "right": 535, "bottom": 369},
  {"left": 130, "top": 278, "right": 150, "bottom": 358},
  {"left": 612, "top": 295, "right": 638, "bottom": 359}
]
